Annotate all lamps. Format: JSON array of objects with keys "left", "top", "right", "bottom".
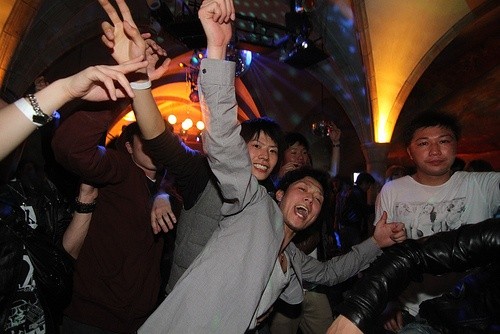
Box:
[
  {"left": 145, "top": 0, "right": 176, "bottom": 31},
  {"left": 311, "top": 84, "right": 333, "bottom": 137},
  {"left": 178, "top": 47, "right": 245, "bottom": 102},
  {"left": 280, "top": 38, "right": 315, "bottom": 64}
]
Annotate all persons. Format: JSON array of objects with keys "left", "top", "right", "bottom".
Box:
[
  {"left": 53, "top": 0, "right": 407, "bottom": 334},
  {"left": 0, "top": 59, "right": 178, "bottom": 334},
  {"left": 327, "top": 111, "right": 500, "bottom": 334}
]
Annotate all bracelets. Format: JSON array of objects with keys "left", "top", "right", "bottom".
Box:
[
  {"left": 15, "top": 93, "right": 53, "bottom": 127},
  {"left": 130, "top": 81, "right": 151, "bottom": 90},
  {"left": 74, "top": 197, "right": 97, "bottom": 213},
  {"left": 332, "top": 144, "right": 341, "bottom": 148}
]
